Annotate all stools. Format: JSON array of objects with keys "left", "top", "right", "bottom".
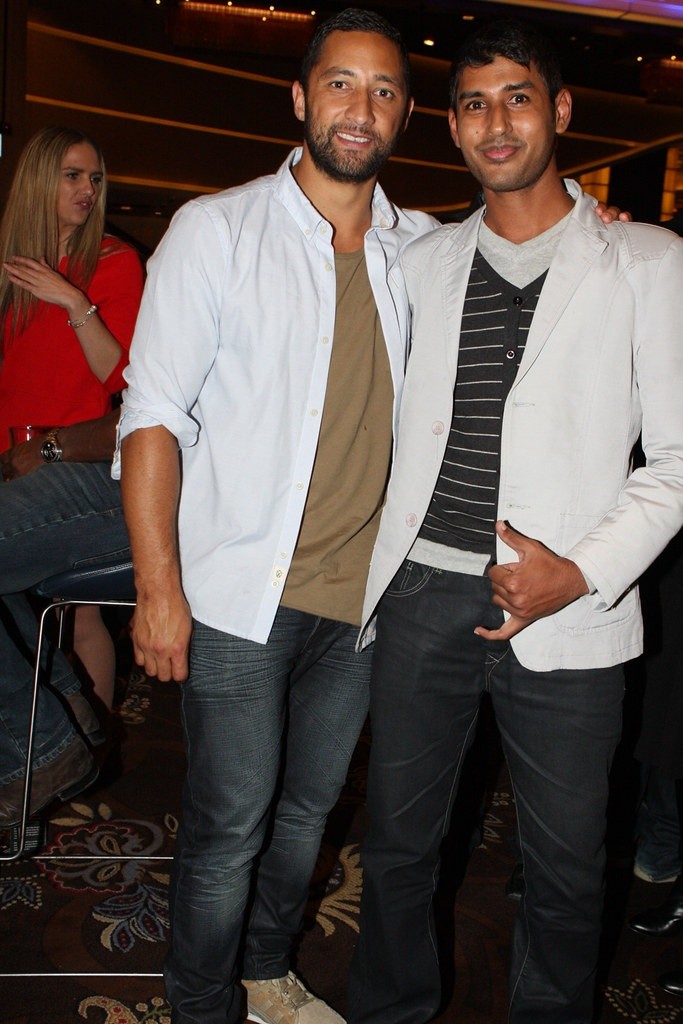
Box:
[{"left": 0, "top": 562, "right": 186, "bottom": 862}]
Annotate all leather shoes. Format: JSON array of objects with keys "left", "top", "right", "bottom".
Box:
[
  {"left": 658, "top": 971, "right": 683, "bottom": 998},
  {"left": 629, "top": 876, "right": 683, "bottom": 938}
]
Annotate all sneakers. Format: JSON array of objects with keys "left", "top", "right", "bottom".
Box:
[{"left": 239, "top": 971, "right": 347, "bottom": 1023}]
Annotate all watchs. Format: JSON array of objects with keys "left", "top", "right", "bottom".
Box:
[{"left": 40, "top": 426, "right": 64, "bottom": 463}]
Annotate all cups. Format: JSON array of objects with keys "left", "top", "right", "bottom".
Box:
[{"left": 9, "top": 425, "right": 53, "bottom": 447}]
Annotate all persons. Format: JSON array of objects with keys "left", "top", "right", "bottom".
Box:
[
  {"left": 109, "top": 2, "right": 632, "bottom": 1024},
  {"left": 344, "top": 18, "right": 683, "bottom": 1024},
  {"left": 0, "top": 117, "right": 146, "bottom": 828}
]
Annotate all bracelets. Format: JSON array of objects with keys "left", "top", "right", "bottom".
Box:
[{"left": 67, "top": 305, "right": 98, "bottom": 328}]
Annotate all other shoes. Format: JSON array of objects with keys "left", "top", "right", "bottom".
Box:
[
  {"left": 0, "top": 734, "right": 101, "bottom": 829},
  {"left": 62, "top": 691, "right": 105, "bottom": 745}
]
